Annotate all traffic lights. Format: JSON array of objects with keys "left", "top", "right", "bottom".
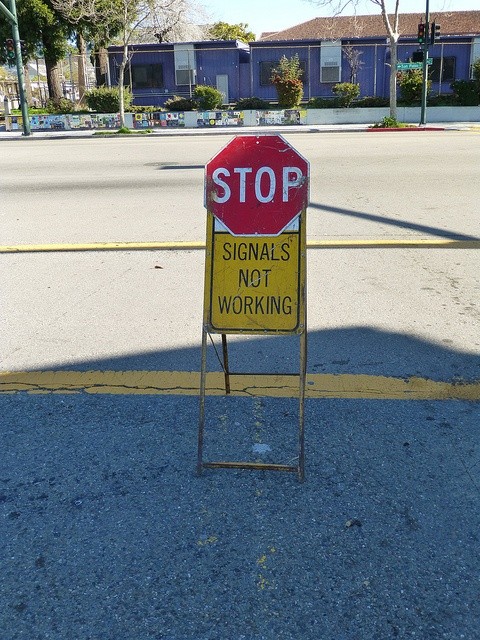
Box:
[
  {"left": 412, "top": 51, "right": 423, "bottom": 62},
  {"left": 418, "top": 24, "right": 424, "bottom": 43},
  {"left": 431, "top": 22, "right": 440, "bottom": 44},
  {"left": 6, "top": 38, "right": 15, "bottom": 59},
  {"left": 20, "top": 40, "right": 28, "bottom": 65}
]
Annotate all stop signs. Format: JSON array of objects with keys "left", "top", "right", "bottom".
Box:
[{"left": 203, "top": 133, "right": 310, "bottom": 237}]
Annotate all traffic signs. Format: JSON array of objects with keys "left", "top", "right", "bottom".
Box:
[
  {"left": 203, "top": 232, "right": 306, "bottom": 335},
  {"left": 428, "top": 58, "right": 433, "bottom": 65},
  {"left": 396, "top": 63, "right": 422, "bottom": 70}
]
